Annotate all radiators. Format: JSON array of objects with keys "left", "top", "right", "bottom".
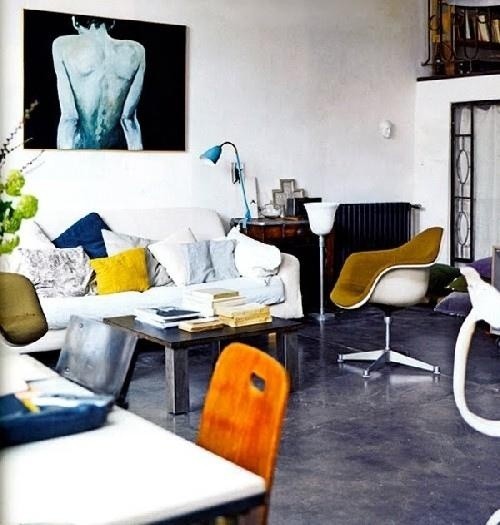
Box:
[{"left": 334, "top": 202, "right": 421, "bottom": 289}]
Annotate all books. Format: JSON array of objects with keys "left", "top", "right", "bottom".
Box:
[{"left": 133, "top": 287, "right": 273, "bottom": 332}]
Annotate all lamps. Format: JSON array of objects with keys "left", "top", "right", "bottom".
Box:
[
  {"left": 199, "top": 141, "right": 251, "bottom": 229},
  {"left": 303, "top": 201, "right": 341, "bottom": 322}
]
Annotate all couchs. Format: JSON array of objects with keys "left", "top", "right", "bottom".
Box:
[{"left": 0, "top": 206, "right": 307, "bottom": 356}]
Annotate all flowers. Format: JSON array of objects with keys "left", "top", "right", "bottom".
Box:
[{"left": 0, "top": 100, "right": 45, "bottom": 255}]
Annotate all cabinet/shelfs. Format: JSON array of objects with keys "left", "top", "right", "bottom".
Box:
[
  {"left": 489, "top": 246, "right": 500, "bottom": 346},
  {"left": 230, "top": 217, "right": 335, "bottom": 317}
]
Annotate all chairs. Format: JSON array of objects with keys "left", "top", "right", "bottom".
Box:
[
  {"left": 54, "top": 312, "right": 141, "bottom": 410},
  {"left": 194, "top": 341, "right": 291, "bottom": 524},
  {"left": 328, "top": 226, "right": 444, "bottom": 381},
  {"left": 452, "top": 266, "right": 500, "bottom": 525}
]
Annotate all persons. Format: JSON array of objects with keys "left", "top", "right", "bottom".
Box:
[{"left": 50, "top": 11, "right": 150, "bottom": 151}]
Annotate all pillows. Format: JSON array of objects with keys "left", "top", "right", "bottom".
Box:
[
  {"left": 445, "top": 274, "right": 469, "bottom": 292},
  {"left": 1, "top": 212, "right": 282, "bottom": 347},
  {"left": 433, "top": 292, "right": 472, "bottom": 318},
  {"left": 465, "top": 256, "right": 492, "bottom": 283}
]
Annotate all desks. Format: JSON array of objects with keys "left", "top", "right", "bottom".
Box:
[{"left": 0, "top": 353, "right": 265, "bottom": 525}]
